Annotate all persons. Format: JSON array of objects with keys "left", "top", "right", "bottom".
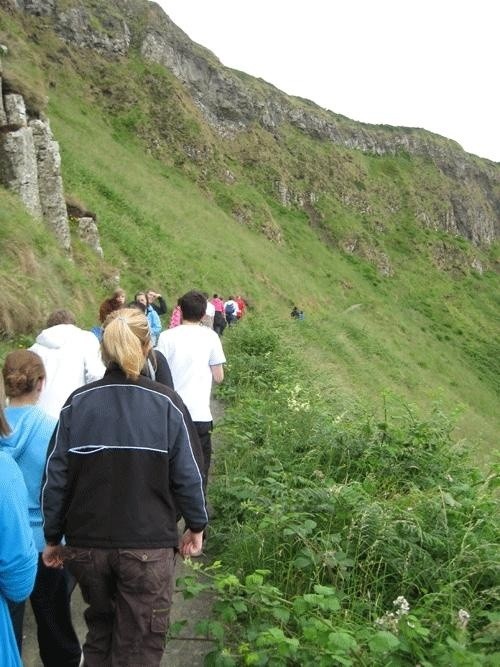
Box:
[
  {"left": 41, "top": 309, "right": 209, "bottom": 666},
  {"left": 157, "top": 288, "right": 250, "bottom": 549},
  {"left": 289, "top": 304, "right": 299, "bottom": 316},
  {"left": 298, "top": 310, "right": 304, "bottom": 319},
  {"left": 27, "top": 290, "right": 175, "bottom": 419},
  {"left": 0, "top": 348, "right": 84, "bottom": 666}
]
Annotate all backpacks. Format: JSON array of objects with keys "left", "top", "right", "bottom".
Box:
[{"left": 226, "top": 302, "right": 234, "bottom": 314}]
